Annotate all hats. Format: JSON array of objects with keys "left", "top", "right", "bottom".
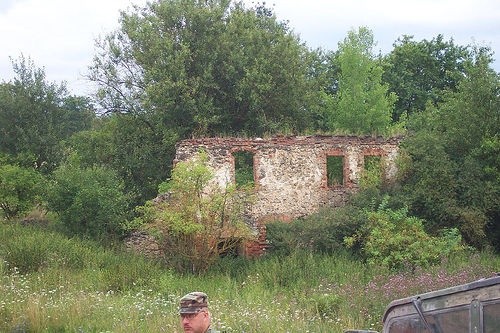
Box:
[{"left": 178, "top": 291, "right": 209, "bottom": 316}]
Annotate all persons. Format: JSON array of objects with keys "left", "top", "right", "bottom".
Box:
[{"left": 179, "top": 291, "right": 220, "bottom": 333}]
